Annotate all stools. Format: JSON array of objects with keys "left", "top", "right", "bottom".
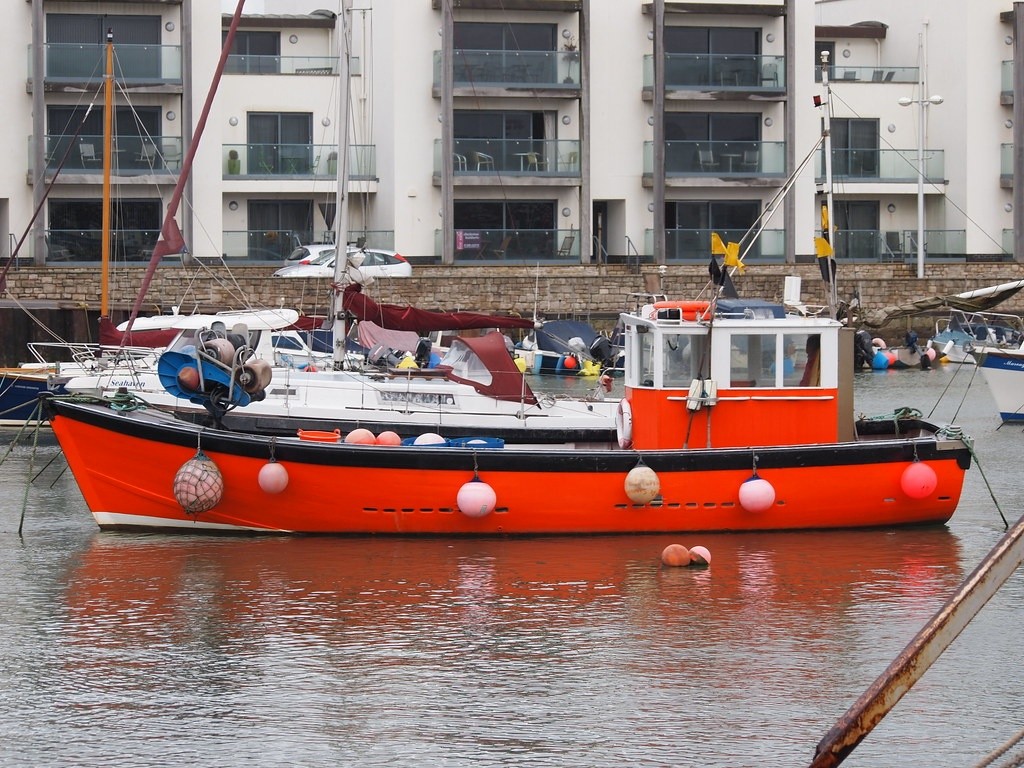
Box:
[{"left": 139, "top": 249, "right": 154, "bottom": 262}]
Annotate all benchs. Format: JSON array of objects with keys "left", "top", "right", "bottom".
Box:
[
  {"left": 295, "top": 68, "right": 332, "bottom": 75},
  {"left": 730, "top": 380, "right": 757, "bottom": 388}
]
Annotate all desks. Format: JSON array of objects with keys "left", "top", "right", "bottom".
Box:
[
  {"left": 719, "top": 153, "right": 742, "bottom": 172},
  {"left": 510, "top": 152, "right": 539, "bottom": 172},
  {"left": 463, "top": 238, "right": 492, "bottom": 259},
  {"left": 280, "top": 156, "right": 304, "bottom": 174}
]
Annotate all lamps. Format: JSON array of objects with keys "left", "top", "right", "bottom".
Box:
[
  {"left": 166, "top": 111, "right": 175, "bottom": 121},
  {"left": 165, "top": 22, "right": 175, "bottom": 33},
  {"left": 887, "top": 204, "right": 896, "bottom": 212},
  {"left": 766, "top": 34, "right": 775, "bottom": 42},
  {"left": 1004, "top": 203, "right": 1013, "bottom": 212},
  {"left": 888, "top": 124, "right": 896, "bottom": 133},
  {"left": 562, "top": 115, "right": 571, "bottom": 125},
  {"left": 229, "top": 201, "right": 237, "bottom": 210},
  {"left": 647, "top": 30, "right": 654, "bottom": 40},
  {"left": 648, "top": 203, "right": 655, "bottom": 212},
  {"left": 562, "top": 207, "right": 571, "bottom": 216},
  {"left": 843, "top": 49, "right": 850, "bottom": 57},
  {"left": 562, "top": 28, "right": 571, "bottom": 39},
  {"left": 229, "top": 117, "right": 238, "bottom": 126},
  {"left": 1005, "top": 35, "right": 1013, "bottom": 45},
  {"left": 764, "top": 117, "right": 773, "bottom": 126},
  {"left": 289, "top": 34, "right": 298, "bottom": 45},
  {"left": 322, "top": 117, "right": 330, "bottom": 126},
  {"left": 647, "top": 116, "right": 653, "bottom": 126},
  {"left": 1005, "top": 119, "right": 1014, "bottom": 129}
]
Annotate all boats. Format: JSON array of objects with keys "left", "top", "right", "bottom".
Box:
[{"left": 0, "top": 0, "right": 1024, "bottom": 534}]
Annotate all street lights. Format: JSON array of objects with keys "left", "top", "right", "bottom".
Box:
[{"left": 895, "top": 96, "right": 944, "bottom": 279}]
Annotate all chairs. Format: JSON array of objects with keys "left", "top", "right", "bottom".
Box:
[
  {"left": 473, "top": 151, "right": 496, "bottom": 172},
  {"left": 798, "top": 350, "right": 821, "bottom": 386},
  {"left": 113, "top": 149, "right": 126, "bottom": 169},
  {"left": 885, "top": 231, "right": 905, "bottom": 263},
  {"left": 842, "top": 69, "right": 895, "bottom": 81},
  {"left": 134, "top": 143, "right": 157, "bottom": 169},
  {"left": 161, "top": 144, "right": 180, "bottom": 170},
  {"left": 555, "top": 151, "right": 577, "bottom": 172},
  {"left": 527, "top": 151, "right": 550, "bottom": 172},
  {"left": 553, "top": 236, "right": 575, "bottom": 257},
  {"left": 305, "top": 155, "right": 319, "bottom": 176},
  {"left": 698, "top": 150, "right": 719, "bottom": 172},
  {"left": 742, "top": 150, "right": 759, "bottom": 172},
  {"left": 79, "top": 143, "right": 102, "bottom": 169},
  {"left": 759, "top": 63, "right": 780, "bottom": 86},
  {"left": 492, "top": 236, "right": 511, "bottom": 260},
  {"left": 255, "top": 150, "right": 272, "bottom": 173},
  {"left": 45, "top": 235, "right": 70, "bottom": 262},
  {"left": 452, "top": 151, "right": 467, "bottom": 172}
]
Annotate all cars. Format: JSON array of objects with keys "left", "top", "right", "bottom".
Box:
[
  {"left": 272, "top": 248, "right": 412, "bottom": 278},
  {"left": 283, "top": 244, "right": 358, "bottom": 266}
]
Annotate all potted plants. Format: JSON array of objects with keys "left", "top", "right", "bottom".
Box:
[
  {"left": 226, "top": 149, "right": 241, "bottom": 175},
  {"left": 264, "top": 231, "right": 279, "bottom": 260},
  {"left": 562, "top": 43, "right": 580, "bottom": 83},
  {"left": 328, "top": 152, "right": 339, "bottom": 175}
]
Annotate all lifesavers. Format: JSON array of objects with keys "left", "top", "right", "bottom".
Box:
[
  {"left": 872, "top": 338, "right": 886, "bottom": 349},
  {"left": 653, "top": 300, "right": 717, "bottom": 320}
]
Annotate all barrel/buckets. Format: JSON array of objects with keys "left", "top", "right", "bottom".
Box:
[{"left": 297, "top": 428, "right": 505, "bottom": 449}]
